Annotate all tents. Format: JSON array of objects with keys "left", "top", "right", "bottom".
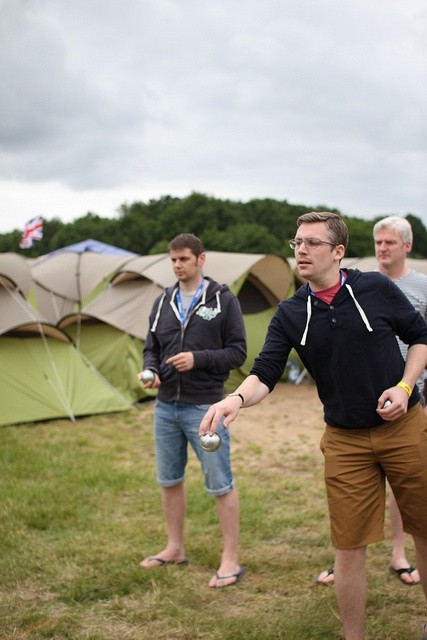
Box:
[{"left": 0, "top": 239, "right": 427, "bottom": 428}]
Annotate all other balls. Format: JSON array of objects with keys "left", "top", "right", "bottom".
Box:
[
  {"left": 142, "top": 369, "right": 155, "bottom": 384},
  {"left": 200, "top": 431, "right": 221, "bottom": 452},
  {"left": 382, "top": 400, "right": 391, "bottom": 409}
]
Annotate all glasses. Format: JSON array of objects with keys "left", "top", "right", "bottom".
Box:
[{"left": 288, "top": 236, "right": 337, "bottom": 252}]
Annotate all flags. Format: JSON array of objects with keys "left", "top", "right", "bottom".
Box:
[{"left": 19, "top": 216, "right": 42, "bottom": 249}]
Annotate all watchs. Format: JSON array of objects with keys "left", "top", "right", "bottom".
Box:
[{"left": 227, "top": 393, "right": 245, "bottom": 408}]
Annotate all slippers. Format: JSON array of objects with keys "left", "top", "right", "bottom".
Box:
[
  {"left": 140, "top": 555, "right": 188, "bottom": 568},
  {"left": 318, "top": 566, "right": 336, "bottom": 586},
  {"left": 209, "top": 568, "right": 243, "bottom": 589},
  {"left": 392, "top": 565, "right": 419, "bottom": 585}
]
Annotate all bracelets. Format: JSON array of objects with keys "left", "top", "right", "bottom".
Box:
[{"left": 397, "top": 381, "right": 412, "bottom": 397}]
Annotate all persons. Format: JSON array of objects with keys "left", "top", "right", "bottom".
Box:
[
  {"left": 317, "top": 213, "right": 427, "bottom": 586},
  {"left": 139, "top": 233, "right": 248, "bottom": 588},
  {"left": 198, "top": 211, "right": 426, "bottom": 640}
]
